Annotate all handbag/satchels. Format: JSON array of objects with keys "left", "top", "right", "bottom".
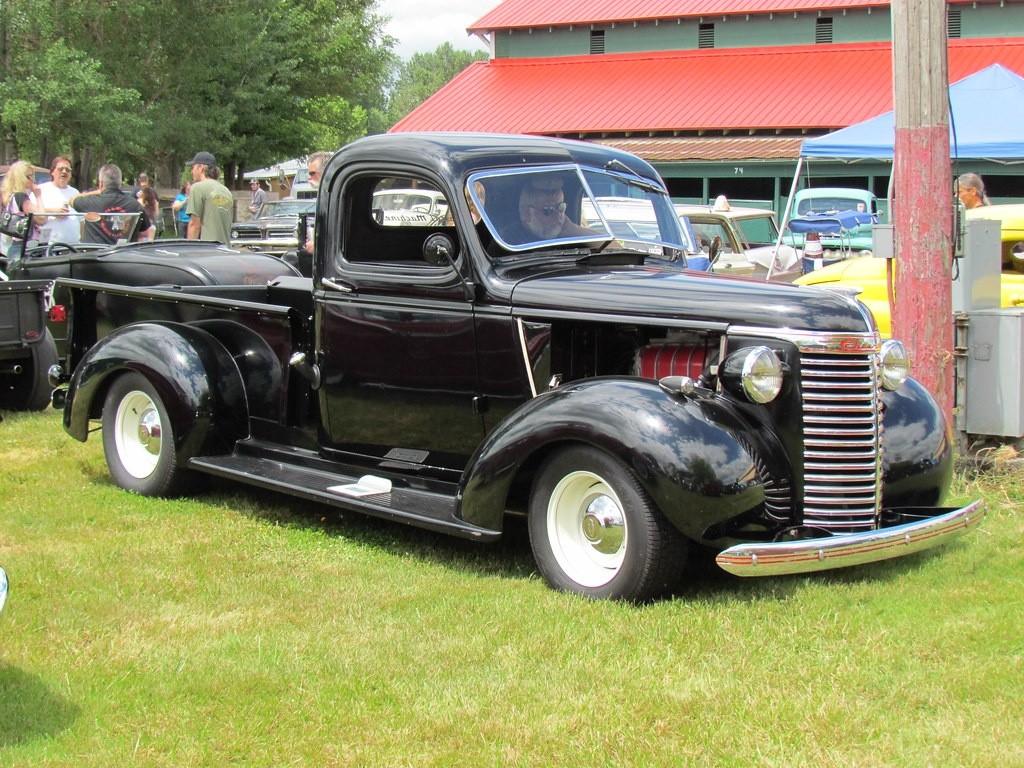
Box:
[{"left": 0, "top": 195, "right": 32, "bottom": 239}]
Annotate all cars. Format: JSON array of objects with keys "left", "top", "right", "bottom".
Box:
[
  {"left": 370, "top": 191, "right": 477, "bottom": 229},
  {"left": 46, "top": 129, "right": 990, "bottom": 610},
  {"left": 0, "top": 210, "right": 305, "bottom": 321},
  {"left": 230, "top": 199, "right": 317, "bottom": 258},
  {"left": 577, "top": 195, "right": 806, "bottom": 288},
  {"left": 788, "top": 204, "right": 1024, "bottom": 342},
  {"left": 779, "top": 188, "right": 886, "bottom": 260},
  {"left": 0, "top": 271, "right": 61, "bottom": 413}
]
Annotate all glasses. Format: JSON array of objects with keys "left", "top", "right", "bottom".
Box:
[
  {"left": 310, "top": 170, "right": 323, "bottom": 176},
  {"left": 524, "top": 202, "right": 566, "bottom": 217},
  {"left": 55, "top": 166, "right": 73, "bottom": 174},
  {"left": 25, "top": 174, "right": 32, "bottom": 179}
]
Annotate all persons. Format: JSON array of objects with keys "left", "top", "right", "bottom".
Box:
[
  {"left": 29, "top": 155, "right": 84, "bottom": 245},
  {"left": 0, "top": 160, "right": 46, "bottom": 282},
  {"left": 247, "top": 178, "right": 267, "bottom": 221},
  {"left": 304, "top": 151, "right": 335, "bottom": 252},
  {"left": 184, "top": 151, "right": 233, "bottom": 246},
  {"left": 134, "top": 173, "right": 159, "bottom": 226},
  {"left": 463, "top": 182, "right": 486, "bottom": 223},
  {"left": 953, "top": 172, "right": 991, "bottom": 209},
  {"left": 70, "top": 163, "right": 154, "bottom": 245},
  {"left": 486, "top": 179, "right": 624, "bottom": 259},
  {"left": 172, "top": 182, "right": 191, "bottom": 238}
]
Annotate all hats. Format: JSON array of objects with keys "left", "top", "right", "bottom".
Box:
[
  {"left": 250, "top": 179, "right": 259, "bottom": 186},
  {"left": 185, "top": 152, "right": 216, "bottom": 167}
]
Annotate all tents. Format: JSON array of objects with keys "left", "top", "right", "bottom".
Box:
[{"left": 766, "top": 63, "right": 1024, "bottom": 281}]
